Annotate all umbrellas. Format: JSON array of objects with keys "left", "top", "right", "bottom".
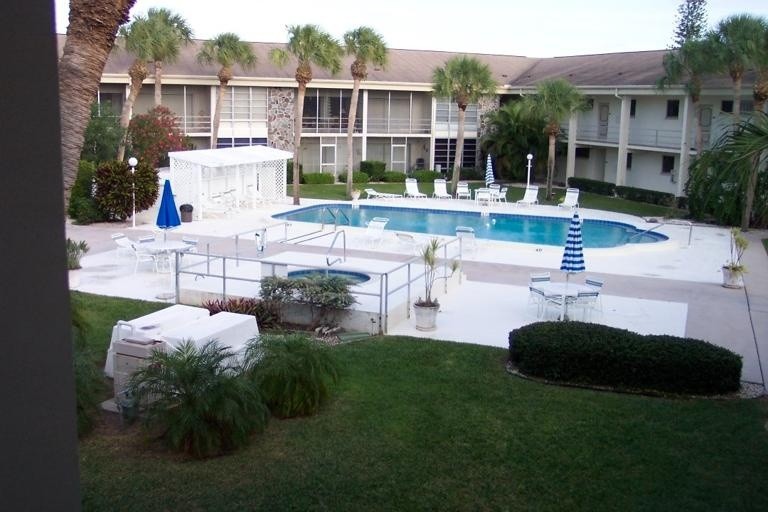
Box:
[
  {"left": 556, "top": 210, "right": 587, "bottom": 285},
  {"left": 152, "top": 178, "right": 182, "bottom": 244},
  {"left": 483, "top": 152, "right": 496, "bottom": 188}
]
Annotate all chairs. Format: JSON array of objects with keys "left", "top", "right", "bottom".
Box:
[
  {"left": 364, "top": 178, "right": 580, "bottom": 211},
  {"left": 347, "top": 217, "right": 415, "bottom": 247},
  {"left": 201, "top": 183, "right": 273, "bottom": 217},
  {"left": 454, "top": 227, "right": 480, "bottom": 259},
  {"left": 111, "top": 232, "right": 198, "bottom": 274},
  {"left": 528, "top": 272, "right": 605, "bottom": 323}
]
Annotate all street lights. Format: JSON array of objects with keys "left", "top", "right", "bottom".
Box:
[
  {"left": 527, "top": 154, "right": 532, "bottom": 186},
  {"left": 128, "top": 157, "right": 138, "bottom": 227}
]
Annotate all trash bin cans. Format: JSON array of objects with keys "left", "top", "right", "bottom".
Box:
[
  {"left": 113, "top": 338, "right": 166, "bottom": 411},
  {"left": 180, "top": 204, "right": 193, "bottom": 222},
  {"left": 416, "top": 158, "right": 424, "bottom": 170},
  {"left": 435, "top": 165, "right": 441, "bottom": 173}
]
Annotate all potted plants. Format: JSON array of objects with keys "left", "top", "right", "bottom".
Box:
[
  {"left": 412, "top": 237, "right": 461, "bottom": 331},
  {"left": 720, "top": 226, "right": 749, "bottom": 289}
]
